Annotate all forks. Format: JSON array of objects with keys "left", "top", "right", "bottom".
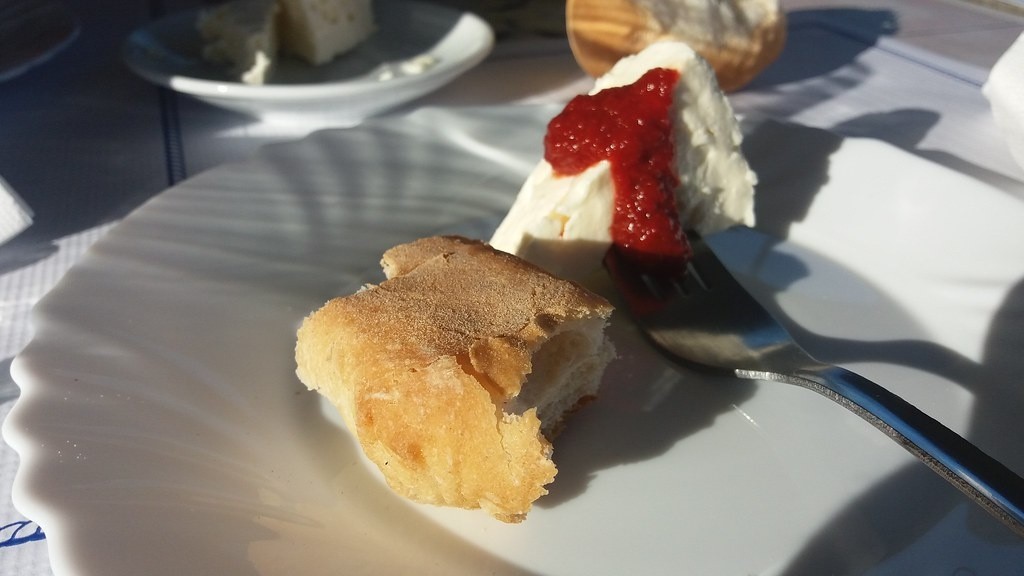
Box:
[{"left": 600, "top": 216, "right": 1024, "bottom": 540}]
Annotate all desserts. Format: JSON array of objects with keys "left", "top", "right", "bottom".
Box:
[
  {"left": 484, "top": 38, "right": 758, "bottom": 318},
  {"left": 194, "top": 0, "right": 377, "bottom": 74}
]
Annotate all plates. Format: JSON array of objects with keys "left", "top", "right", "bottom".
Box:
[
  {"left": 124, "top": 0, "right": 497, "bottom": 130},
  {"left": 2, "top": 102, "right": 1024, "bottom": 576},
  {"left": 0, "top": 0, "right": 81, "bottom": 85}
]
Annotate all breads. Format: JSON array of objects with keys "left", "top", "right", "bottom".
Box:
[
  {"left": 293, "top": 233, "right": 618, "bottom": 523},
  {"left": 563, "top": 0, "right": 789, "bottom": 97}
]
